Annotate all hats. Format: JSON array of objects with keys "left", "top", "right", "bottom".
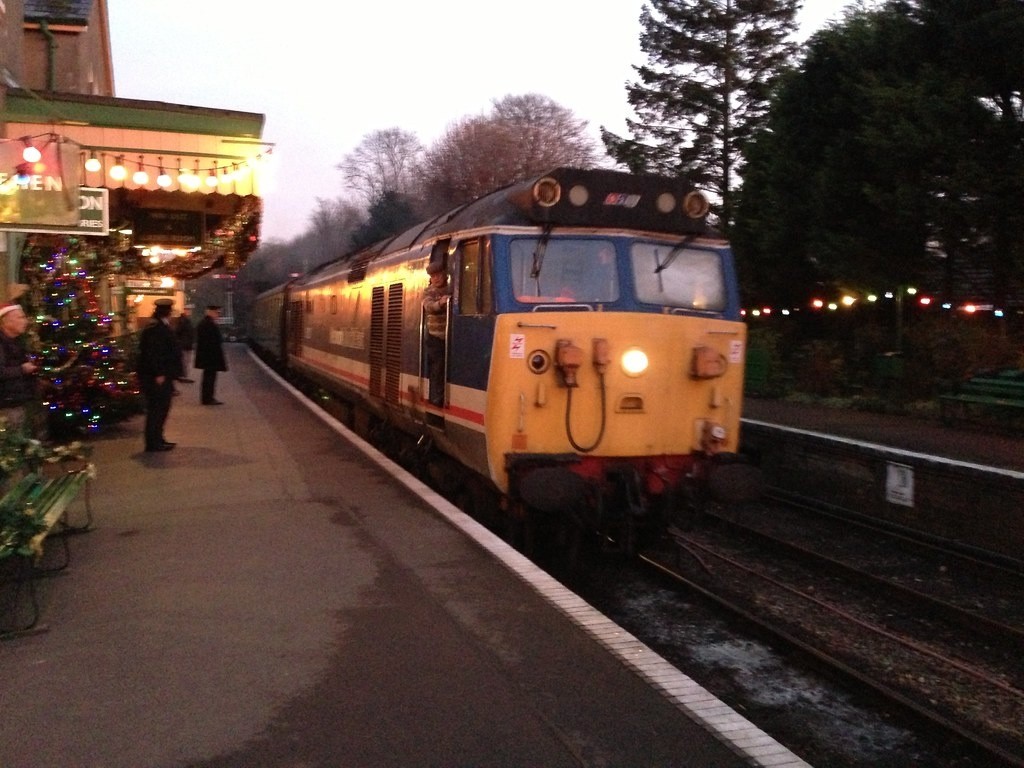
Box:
[
  {"left": 207, "top": 305, "right": 217, "bottom": 311},
  {"left": 0, "top": 303, "right": 21, "bottom": 320},
  {"left": 183, "top": 305, "right": 195, "bottom": 309},
  {"left": 154, "top": 299, "right": 174, "bottom": 307}
]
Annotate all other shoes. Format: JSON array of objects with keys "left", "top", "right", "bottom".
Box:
[
  {"left": 146, "top": 440, "right": 176, "bottom": 451},
  {"left": 203, "top": 399, "right": 223, "bottom": 405}
]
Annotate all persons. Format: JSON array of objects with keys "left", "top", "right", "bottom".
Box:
[
  {"left": 176, "top": 305, "right": 195, "bottom": 382},
  {"left": 421, "top": 263, "right": 452, "bottom": 408},
  {"left": 0, "top": 304, "right": 40, "bottom": 430},
  {"left": 193, "top": 303, "right": 229, "bottom": 405},
  {"left": 139, "top": 299, "right": 184, "bottom": 451}
]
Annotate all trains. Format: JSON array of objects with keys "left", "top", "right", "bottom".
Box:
[{"left": 247, "top": 166, "right": 747, "bottom": 518}]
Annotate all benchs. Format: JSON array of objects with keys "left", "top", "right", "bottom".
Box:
[
  {"left": 1, "top": 416, "right": 93, "bottom": 636},
  {"left": 938, "top": 377, "right": 1024, "bottom": 437}
]
